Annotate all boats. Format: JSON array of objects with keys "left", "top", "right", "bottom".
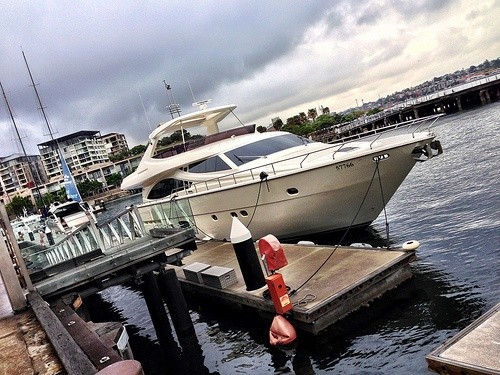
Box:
[
  {"left": 121, "top": 74, "right": 448, "bottom": 245},
  {"left": 0, "top": 43, "right": 102, "bottom": 235}
]
